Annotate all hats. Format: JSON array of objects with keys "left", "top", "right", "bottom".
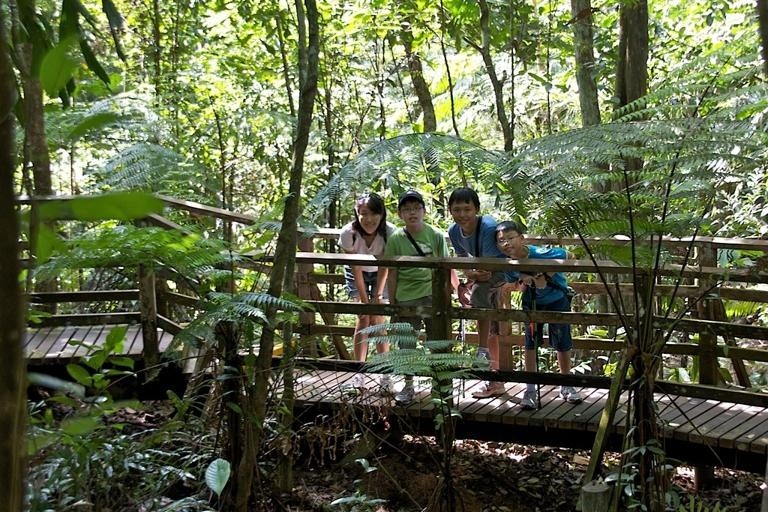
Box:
[{"left": 398, "top": 190, "right": 422, "bottom": 207}]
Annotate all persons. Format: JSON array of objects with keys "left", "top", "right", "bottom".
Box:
[
  {"left": 494, "top": 220, "right": 590, "bottom": 411},
  {"left": 380, "top": 189, "right": 458, "bottom": 405},
  {"left": 337, "top": 191, "right": 398, "bottom": 397},
  {"left": 447, "top": 187, "right": 523, "bottom": 399}
]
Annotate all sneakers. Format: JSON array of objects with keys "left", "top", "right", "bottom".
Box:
[
  {"left": 520, "top": 390, "right": 537, "bottom": 409},
  {"left": 352, "top": 373, "right": 365, "bottom": 389},
  {"left": 471, "top": 351, "right": 491, "bottom": 371},
  {"left": 471, "top": 381, "right": 505, "bottom": 398},
  {"left": 395, "top": 386, "right": 416, "bottom": 404},
  {"left": 560, "top": 385, "right": 582, "bottom": 404},
  {"left": 378, "top": 377, "right": 395, "bottom": 395}
]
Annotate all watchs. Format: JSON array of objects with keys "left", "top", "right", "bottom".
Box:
[
  {"left": 543, "top": 271, "right": 553, "bottom": 283},
  {"left": 372, "top": 294, "right": 385, "bottom": 301}
]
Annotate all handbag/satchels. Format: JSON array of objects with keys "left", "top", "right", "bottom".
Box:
[{"left": 531, "top": 272, "right": 572, "bottom": 311}]
